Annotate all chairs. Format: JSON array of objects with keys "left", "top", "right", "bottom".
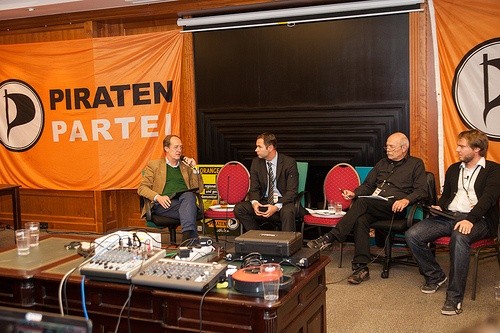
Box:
[
  {"left": 259, "top": 162, "right": 312, "bottom": 228},
  {"left": 301, "top": 162, "right": 361, "bottom": 268},
  {"left": 140, "top": 161, "right": 250, "bottom": 245},
  {"left": 370, "top": 172, "right": 436, "bottom": 278},
  {"left": 429, "top": 223, "right": 500, "bottom": 300}
]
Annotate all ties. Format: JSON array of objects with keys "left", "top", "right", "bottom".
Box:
[{"left": 267, "top": 163, "right": 273, "bottom": 205}]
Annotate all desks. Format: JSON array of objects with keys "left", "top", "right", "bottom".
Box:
[
  {"left": 0, "top": 184, "right": 22, "bottom": 244},
  {"left": 0, "top": 234, "right": 332, "bottom": 333}
]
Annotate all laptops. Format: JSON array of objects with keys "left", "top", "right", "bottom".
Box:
[{"left": 0, "top": 306, "right": 93, "bottom": 333}]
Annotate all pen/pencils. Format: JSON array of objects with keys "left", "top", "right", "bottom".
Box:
[{"left": 338, "top": 187, "right": 350, "bottom": 199}]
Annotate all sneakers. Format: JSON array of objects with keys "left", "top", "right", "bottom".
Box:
[
  {"left": 348, "top": 268, "right": 370, "bottom": 284},
  {"left": 307, "top": 235, "right": 331, "bottom": 252},
  {"left": 441, "top": 288, "right": 463, "bottom": 315},
  {"left": 421, "top": 274, "right": 448, "bottom": 294}
]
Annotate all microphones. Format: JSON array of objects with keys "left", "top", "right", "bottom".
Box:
[{"left": 180, "top": 154, "right": 196, "bottom": 169}]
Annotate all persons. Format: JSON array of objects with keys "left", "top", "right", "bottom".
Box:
[
  {"left": 235, "top": 132, "right": 299, "bottom": 232},
  {"left": 404, "top": 128, "right": 500, "bottom": 315},
  {"left": 306, "top": 132, "right": 429, "bottom": 284},
  {"left": 137, "top": 135, "right": 204, "bottom": 248}
]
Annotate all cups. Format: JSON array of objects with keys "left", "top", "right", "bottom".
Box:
[
  {"left": 328, "top": 203, "right": 336, "bottom": 215},
  {"left": 335, "top": 202, "right": 342, "bottom": 214},
  {"left": 260, "top": 263, "right": 281, "bottom": 301},
  {"left": 495, "top": 281, "right": 500, "bottom": 301},
  {"left": 25, "top": 222, "right": 40, "bottom": 246},
  {"left": 15, "top": 229, "right": 30, "bottom": 255}
]
